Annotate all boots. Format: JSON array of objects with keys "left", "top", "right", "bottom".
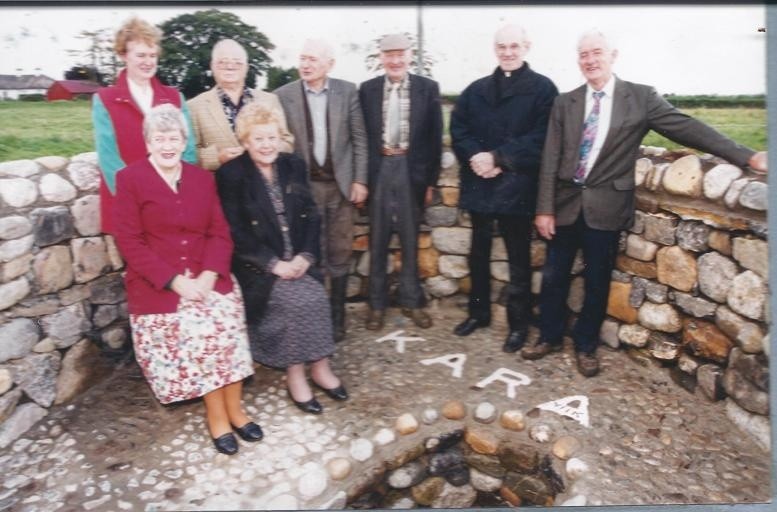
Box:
[{"left": 330, "top": 274, "right": 350, "bottom": 344}]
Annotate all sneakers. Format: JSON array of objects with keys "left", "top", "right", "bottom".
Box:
[
  {"left": 522, "top": 337, "right": 563, "bottom": 360},
  {"left": 577, "top": 350, "right": 600, "bottom": 377}
]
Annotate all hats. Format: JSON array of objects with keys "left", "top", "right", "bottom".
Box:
[{"left": 381, "top": 34, "right": 410, "bottom": 51}]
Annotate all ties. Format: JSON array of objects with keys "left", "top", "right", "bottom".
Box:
[
  {"left": 388, "top": 82, "right": 400, "bottom": 146},
  {"left": 572, "top": 91, "right": 605, "bottom": 182}
]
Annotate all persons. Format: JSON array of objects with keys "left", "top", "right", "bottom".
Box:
[
  {"left": 214, "top": 102, "right": 348, "bottom": 413},
  {"left": 185, "top": 37, "right": 296, "bottom": 177},
  {"left": 359, "top": 34, "right": 445, "bottom": 332},
  {"left": 112, "top": 103, "right": 262, "bottom": 453},
  {"left": 520, "top": 34, "right": 766, "bottom": 377},
  {"left": 449, "top": 23, "right": 559, "bottom": 354},
  {"left": 272, "top": 41, "right": 369, "bottom": 345},
  {"left": 92, "top": 16, "right": 198, "bottom": 239}
]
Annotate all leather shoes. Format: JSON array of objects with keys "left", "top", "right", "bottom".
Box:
[
  {"left": 502, "top": 329, "right": 525, "bottom": 353},
  {"left": 453, "top": 318, "right": 490, "bottom": 336},
  {"left": 288, "top": 389, "right": 322, "bottom": 414},
  {"left": 313, "top": 381, "right": 347, "bottom": 402},
  {"left": 213, "top": 432, "right": 237, "bottom": 456},
  {"left": 402, "top": 306, "right": 431, "bottom": 328},
  {"left": 230, "top": 421, "right": 263, "bottom": 442},
  {"left": 366, "top": 309, "right": 384, "bottom": 331}
]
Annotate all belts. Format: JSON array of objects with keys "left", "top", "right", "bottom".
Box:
[{"left": 382, "top": 148, "right": 406, "bottom": 157}]
